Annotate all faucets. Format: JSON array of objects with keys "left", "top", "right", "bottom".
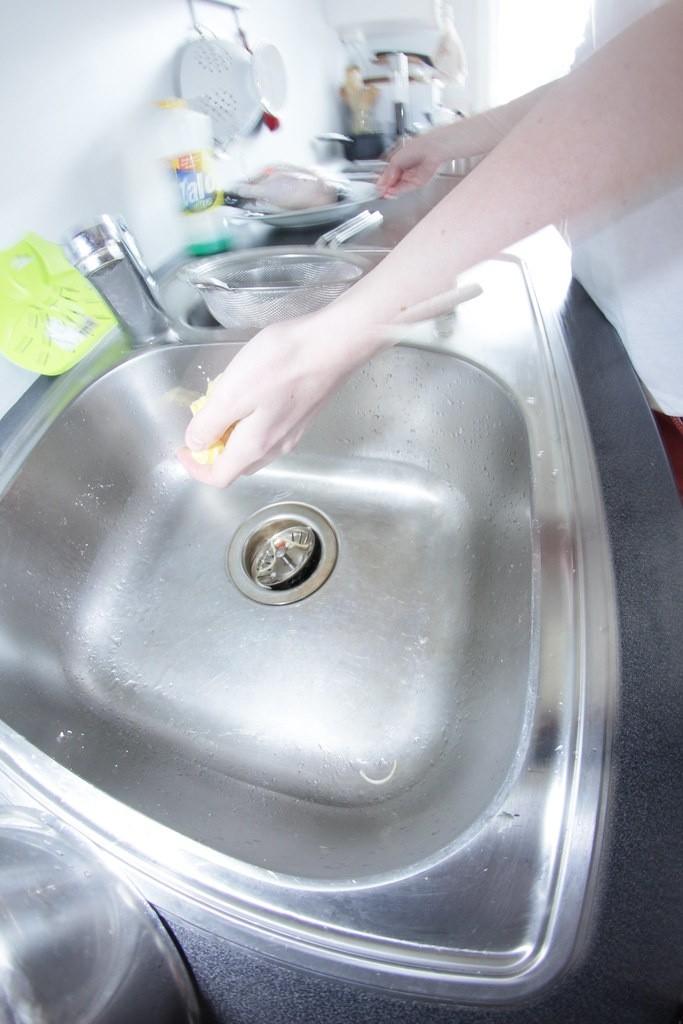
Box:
[{"left": 62, "top": 213, "right": 177, "bottom": 342}]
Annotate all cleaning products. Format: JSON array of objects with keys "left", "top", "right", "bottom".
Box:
[{"left": 145, "top": 94, "right": 233, "bottom": 258}]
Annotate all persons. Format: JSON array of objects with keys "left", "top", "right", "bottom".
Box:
[{"left": 181, "top": 1, "right": 683, "bottom": 511}]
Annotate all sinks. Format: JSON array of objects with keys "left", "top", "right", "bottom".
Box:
[
  {"left": 0, "top": 344, "right": 625, "bottom": 1012},
  {"left": 176, "top": 245, "right": 400, "bottom": 333}
]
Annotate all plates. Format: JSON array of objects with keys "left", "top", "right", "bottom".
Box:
[{"left": 235, "top": 181, "right": 384, "bottom": 228}]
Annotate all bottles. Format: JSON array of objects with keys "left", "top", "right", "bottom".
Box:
[{"left": 150, "top": 100, "right": 228, "bottom": 253}]
